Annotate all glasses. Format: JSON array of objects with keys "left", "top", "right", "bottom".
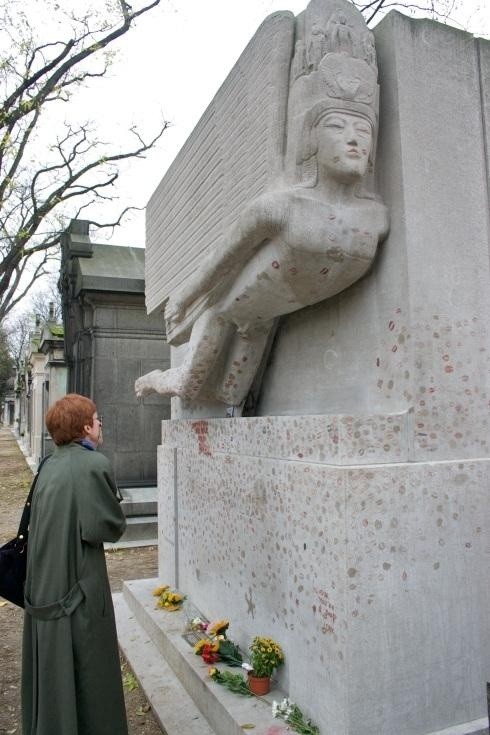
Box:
[{"left": 92, "top": 415, "right": 103, "bottom": 423}]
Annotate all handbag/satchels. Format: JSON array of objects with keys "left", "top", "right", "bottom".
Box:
[{"left": 0, "top": 531, "right": 30, "bottom": 610}]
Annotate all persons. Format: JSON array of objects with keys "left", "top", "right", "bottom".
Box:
[
  {"left": 291, "top": 12, "right": 379, "bottom": 78},
  {"left": 20, "top": 395, "right": 125, "bottom": 735},
  {"left": 132, "top": 49, "right": 388, "bottom": 404}
]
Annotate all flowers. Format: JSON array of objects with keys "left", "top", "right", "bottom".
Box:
[{"left": 152, "top": 583, "right": 322, "bottom": 735}]
